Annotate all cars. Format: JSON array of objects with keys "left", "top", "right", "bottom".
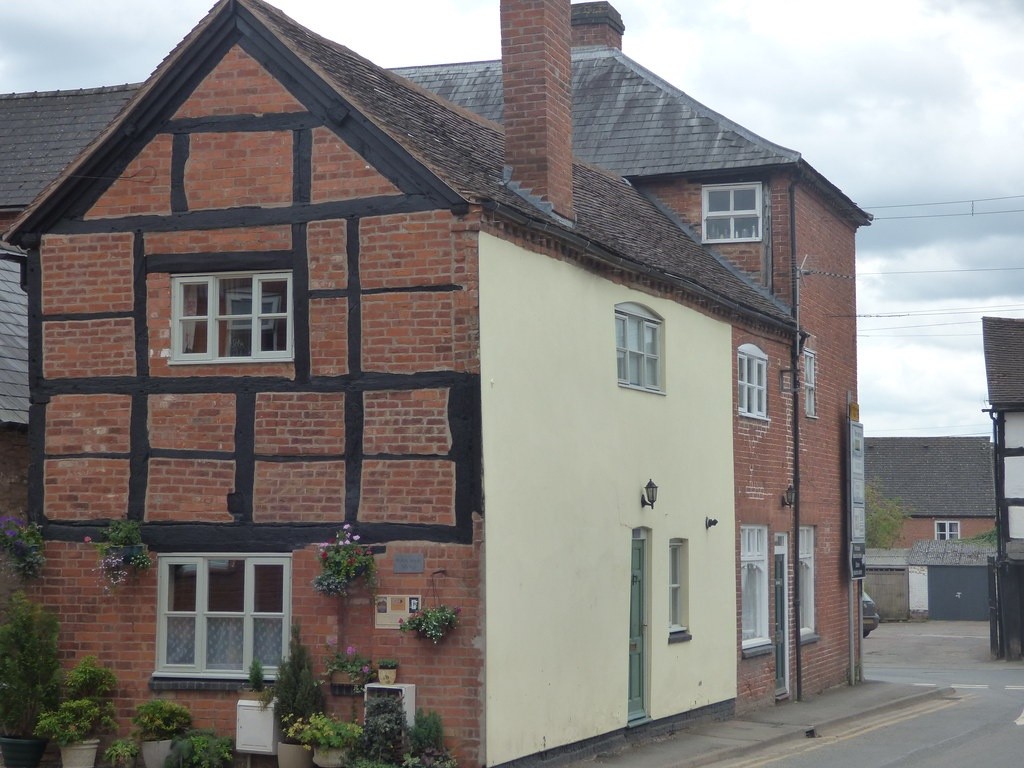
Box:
[{"left": 861, "top": 592, "right": 879, "bottom": 637}]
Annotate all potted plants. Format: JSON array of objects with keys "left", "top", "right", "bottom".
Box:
[
  {"left": 34, "top": 654, "right": 119, "bottom": 768},
  {"left": 281, "top": 712, "right": 363, "bottom": 768},
  {"left": 1, "top": 589, "right": 64, "bottom": 768},
  {"left": 105, "top": 739, "right": 139, "bottom": 768},
  {"left": 129, "top": 698, "right": 192, "bottom": 768},
  {"left": 248, "top": 657, "right": 266, "bottom": 700},
  {"left": 378, "top": 658, "right": 398, "bottom": 684},
  {"left": 273, "top": 621, "right": 325, "bottom": 768}
]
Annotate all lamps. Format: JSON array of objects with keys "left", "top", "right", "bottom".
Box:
[
  {"left": 641, "top": 479, "right": 657, "bottom": 510},
  {"left": 782, "top": 485, "right": 796, "bottom": 508}
]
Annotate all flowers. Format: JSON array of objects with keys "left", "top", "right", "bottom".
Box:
[
  {"left": 85, "top": 519, "right": 151, "bottom": 595},
  {"left": 398, "top": 607, "right": 461, "bottom": 644},
  {"left": 319, "top": 524, "right": 373, "bottom": 580},
  {"left": 321, "top": 643, "right": 376, "bottom": 693},
  {"left": 1, "top": 515, "right": 46, "bottom": 584}
]
